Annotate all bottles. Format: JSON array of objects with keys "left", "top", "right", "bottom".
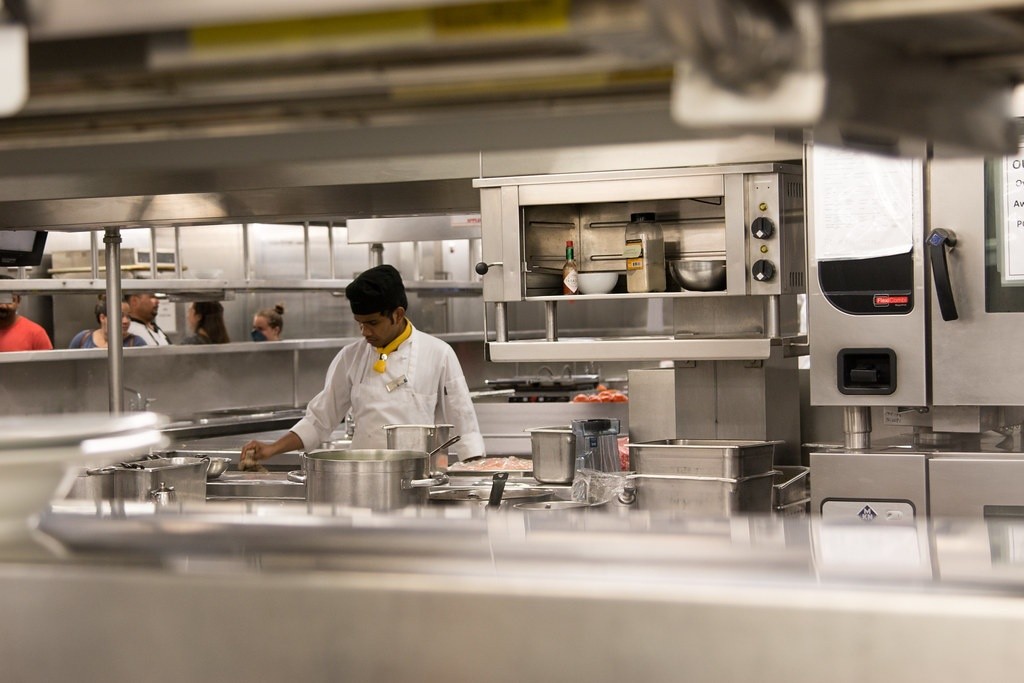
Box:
[{"left": 562, "top": 241, "right": 577, "bottom": 295}]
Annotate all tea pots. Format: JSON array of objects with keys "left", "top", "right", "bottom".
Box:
[{"left": 151, "top": 482, "right": 176, "bottom": 503}]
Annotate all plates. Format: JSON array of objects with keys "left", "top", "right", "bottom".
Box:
[{"left": 447, "top": 469, "right": 533, "bottom": 477}]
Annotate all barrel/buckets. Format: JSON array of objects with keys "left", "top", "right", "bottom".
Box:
[
  {"left": 514, "top": 501, "right": 590, "bottom": 534},
  {"left": 382, "top": 424, "right": 454, "bottom": 477},
  {"left": 523, "top": 426, "right": 576, "bottom": 483}
]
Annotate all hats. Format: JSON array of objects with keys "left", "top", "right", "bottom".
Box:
[{"left": 345, "top": 264, "right": 407, "bottom": 316}]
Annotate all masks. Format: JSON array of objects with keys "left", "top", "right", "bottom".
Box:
[{"left": 250, "top": 329, "right": 268, "bottom": 342}]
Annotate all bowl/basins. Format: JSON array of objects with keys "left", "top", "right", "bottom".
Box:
[
  {"left": 669, "top": 261, "right": 727, "bottom": 292},
  {"left": 577, "top": 273, "right": 619, "bottom": 294},
  {"left": 207, "top": 457, "right": 232, "bottom": 479}
]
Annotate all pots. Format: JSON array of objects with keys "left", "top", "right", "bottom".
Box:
[
  {"left": 429, "top": 472, "right": 553, "bottom": 511},
  {"left": 305, "top": 449, "right": 449, "bottom": 508}
]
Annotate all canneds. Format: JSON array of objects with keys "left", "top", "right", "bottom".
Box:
[{"left": 624, "top": 212, "right": 666, "bottom": 292}]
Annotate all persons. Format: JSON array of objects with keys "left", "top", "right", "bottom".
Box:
[
  {"left": 248, "top": 303, "right": 284, "bottom": 341},
  {"left": 239, "top": 263, "right": 487, "bottom": 472},
  {"left": 65, "top": 292, "right": 146, "bottom": 348},
  {"left": 178, "top": 300, "right": 230, "bottom": 344},
  {"left": 120, "top": 292, "right": 172, "bottom": 346},
  {"left": 0, "top": 274, "right": 54, "bottom": 351}
]
